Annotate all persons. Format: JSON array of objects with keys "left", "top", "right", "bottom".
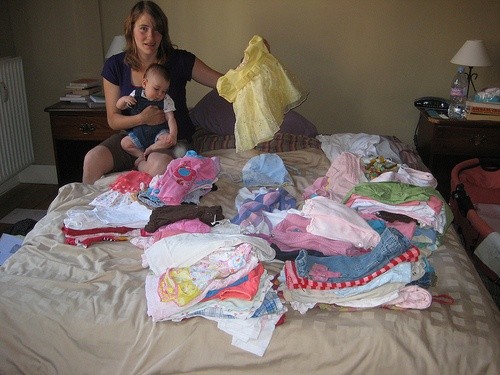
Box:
[
  {"left": 116, "top": 63, "right": 177, "bottom": 165},
  {"left": 82, "top": 0, "right": 225, "bottom": 185}
]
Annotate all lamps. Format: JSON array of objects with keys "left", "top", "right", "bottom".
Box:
[{"left": 450, "top": 40, "right": 493, "bottom": 96}]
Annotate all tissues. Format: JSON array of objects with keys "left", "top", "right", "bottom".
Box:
[{"left": 473, "top": 87, "right": 500, "bottom": 103}]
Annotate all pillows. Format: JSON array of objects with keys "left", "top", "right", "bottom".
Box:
[{"left": 190, "top": 89, "right": 319, "bottom": 138}]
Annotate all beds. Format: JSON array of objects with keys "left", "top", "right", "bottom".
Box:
[{"left": 0, "top": 137, "right": 500, "bottom": 375}]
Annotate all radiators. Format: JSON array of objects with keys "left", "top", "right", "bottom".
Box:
[{"left": 0, "top": 56, "right": 35, "bottom": 185}]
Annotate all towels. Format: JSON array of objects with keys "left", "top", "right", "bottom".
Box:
[
  {"left": 451, "top": 183, "right": 476, "bottom": 219},
  {"left": 473, "top": 231, "right": 500, "bottom": 279}
]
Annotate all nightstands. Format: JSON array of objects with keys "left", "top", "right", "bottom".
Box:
[
  {"left": 44, "top": 101, "right": 122, "bottom": 188},
  {"left": 417, "top": 110, "right": 500, "bottom": 200}
]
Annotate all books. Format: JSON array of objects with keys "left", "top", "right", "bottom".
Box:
[
  {"left": 465, "top": 99, "right": 500, "bottom": 121},
  {"left": 59, "top": 79, "right": 106, "bottom": 108}
]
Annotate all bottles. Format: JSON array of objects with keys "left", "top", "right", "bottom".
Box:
[{"left": 447, "top": 66, "right": 468, "bottom": 119}]
